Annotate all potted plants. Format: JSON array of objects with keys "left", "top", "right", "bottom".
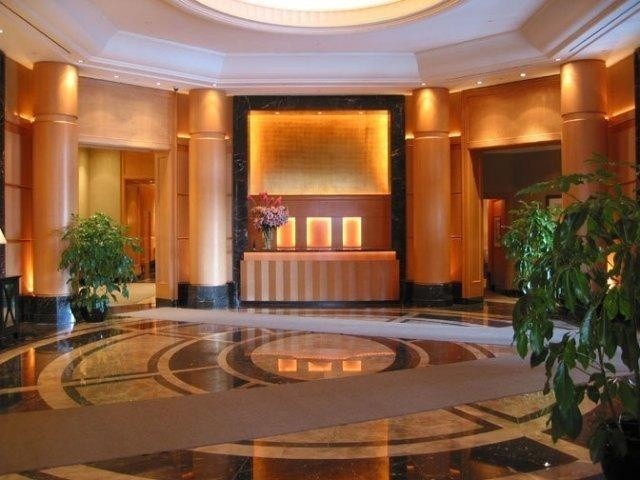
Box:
[
  {"left": 491, "top": 147, "right": 640, "bottom": 480},
  {"left": 47, "top": 207, "right": 147, "bottom": 322}
]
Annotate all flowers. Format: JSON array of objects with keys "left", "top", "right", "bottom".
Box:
[{"left": 244, "top": 189, "right": 294, "bottom": 234}]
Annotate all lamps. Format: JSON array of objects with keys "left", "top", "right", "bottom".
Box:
[{"left": 0, "top": 228, "right": 8, "bottom": 245}]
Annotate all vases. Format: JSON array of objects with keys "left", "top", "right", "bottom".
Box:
[{"left": 258, "top": 223, "right": 277, "bottom": 252}]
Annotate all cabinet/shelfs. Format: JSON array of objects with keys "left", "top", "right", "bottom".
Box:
[{"left": 0, "top": 271, "right": 24, "bottom": 352}]
